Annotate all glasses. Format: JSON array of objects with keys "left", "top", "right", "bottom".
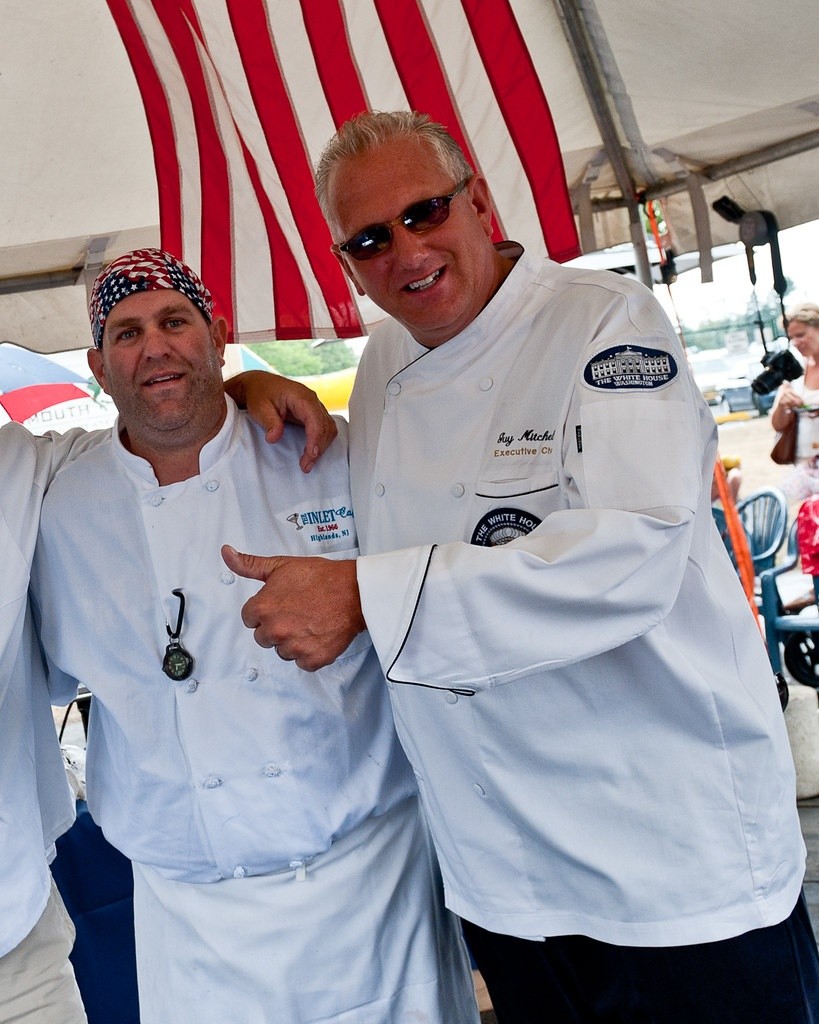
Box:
[{"left": 338, "top": 176, "right": 473, "bottom": 260}]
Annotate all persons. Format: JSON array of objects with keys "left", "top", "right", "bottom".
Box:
[
  {"left": 24, "top": 249, "right": 482, "bottom": 1024},
  {"left": 3, "top": 367, "right": 337, "bottom": 1024},
  {"left": 218, "top": 106, "right": 819, "bottom": 1024},
  {"left": 768, "top": 305, "right": 819, "bottom": 508}
]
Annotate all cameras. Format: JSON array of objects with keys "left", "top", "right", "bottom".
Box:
[{"left": 751, "top": 349, "right": 803, "bottom": 396}]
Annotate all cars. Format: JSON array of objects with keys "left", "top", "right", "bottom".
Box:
[{"left": 681, "top": 334, "right": 788, "bottom": 417}]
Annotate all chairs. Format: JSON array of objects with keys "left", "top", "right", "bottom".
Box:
[{"left": 711, "top": 489, "right": 819, "bottom": 676}]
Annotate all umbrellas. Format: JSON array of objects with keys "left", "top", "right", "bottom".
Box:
[{"left": 1, "top": 340, "right": 95, "bottom": 427}]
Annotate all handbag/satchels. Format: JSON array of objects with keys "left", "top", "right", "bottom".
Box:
[{"left": 770, "top": 412, "right": 800, "bottom": 465}]
